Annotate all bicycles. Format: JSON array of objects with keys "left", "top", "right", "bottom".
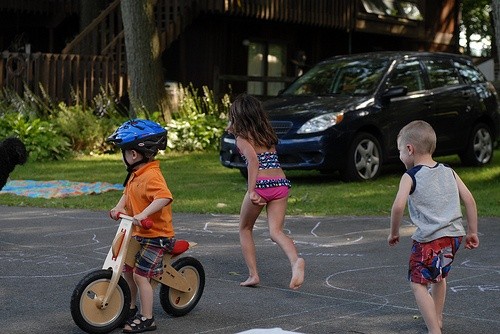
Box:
[{"left": 68, "top": 212, "right": 207, "bottom": 334}]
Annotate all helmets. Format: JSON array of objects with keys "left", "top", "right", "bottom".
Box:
[{"left": 104, "top": 121, "right": 169, "bottom": 154}]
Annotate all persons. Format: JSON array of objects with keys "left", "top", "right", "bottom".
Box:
[
  {"left": 106, "top": 118, "right": 176, "bottom": 333},
  {"left": 386, "top": 121, "right": 480, "bottom": 334},
  {"left": 227, "top": 98, "right": 305, "bottom": 289}
]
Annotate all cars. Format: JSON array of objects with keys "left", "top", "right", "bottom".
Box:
[{"left": 218, "top": 51, "right": 500, "bottom": 184}]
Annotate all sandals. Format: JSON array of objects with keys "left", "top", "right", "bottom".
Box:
[
  {"left": 124, "top": 306, "right": 139, "bottom": 322},
  {"left": 123, "top": 314, "right": 157, "bottom": 332}
]
